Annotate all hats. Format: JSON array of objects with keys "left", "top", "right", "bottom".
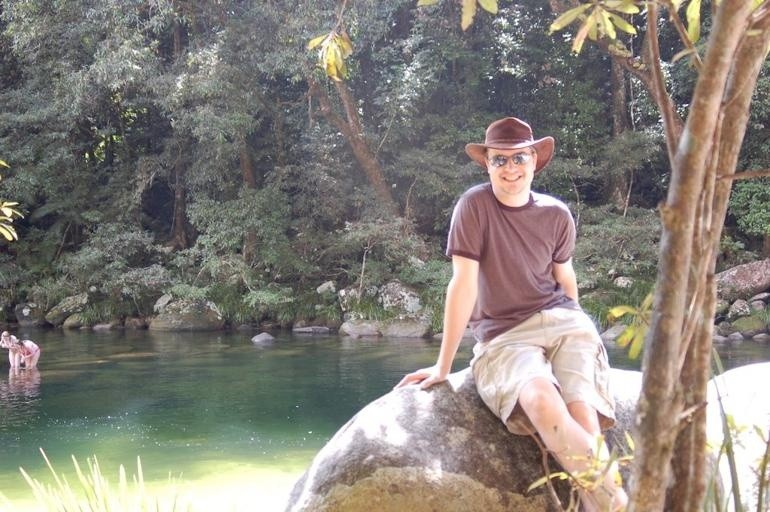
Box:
[{"left": 465, "top": 117, "right": 554, "bottom": 176}]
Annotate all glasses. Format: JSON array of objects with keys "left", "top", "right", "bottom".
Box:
[{"left": 485, "top": 153, "right": 535, "bottom": 166}]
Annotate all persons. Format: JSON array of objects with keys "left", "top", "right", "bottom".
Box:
[
  {"left": 0, "top": 330, "right": 20, "bottom": 368},
  {"left": 14, "top": 339, "right": 40, "bottom": 368},
  {"left": 9, "top": 367, "right": 20, "bottom": 392},
  {"left": 392, "top": 118, "right": 629, "bottom": 512},
  {"left": 26, "top": 366, "right": 40, "bottom": 397}
]
